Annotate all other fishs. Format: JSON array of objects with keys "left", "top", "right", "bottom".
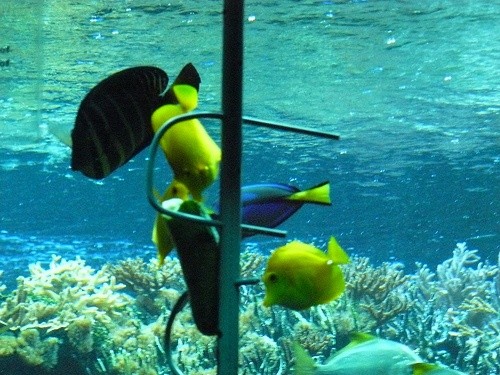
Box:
[
  {"left": 166, "top": 198, "right": 222, "bottom": 337},
  {"left": 215, "top": 180, "right": 334, "bottom": 240},
  {"left": 258, "top": 236, "right": 351, "bottom": 312},
  {"left": 151, "top": 84, "right": 222, "bottom": 204},
  {"left": 149, "top": 179, "right": 191, "bottom": 270},
  {"left": 70, "top": 63, "right": 203, "bottom": 180},
  {"left": 292, "top": 331, "right": 424, "bottom": 375},
  {"left": 410, "top": 363, "right": 468, "bottom": 375}
]
